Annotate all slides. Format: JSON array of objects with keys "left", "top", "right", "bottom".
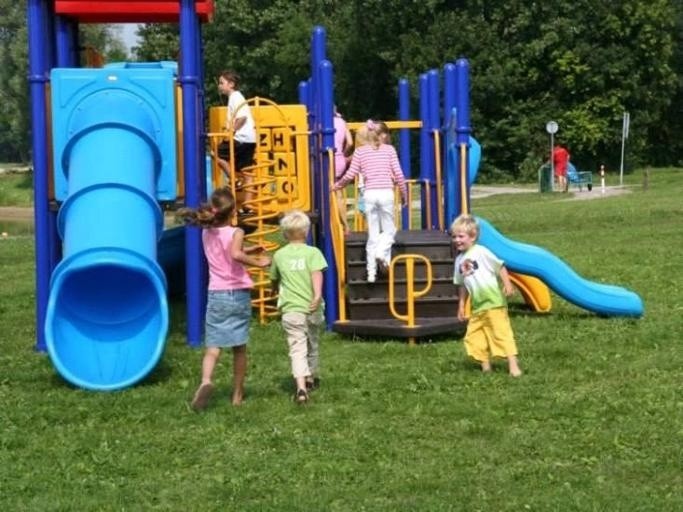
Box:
[
  {"left": 475, "top": 217, "right": 643, "bottom": 316},
  {"left": 44, "top": 68, "right": 179, "bottom": 391}
]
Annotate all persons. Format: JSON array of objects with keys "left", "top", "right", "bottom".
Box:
[
  {"left": 448, "top": 212, "right": 523, "bottom": 378},
  {"left": 354, "top": 124, "right": 370, "bottom": 219},
  {"left": 552, "top": 142, "right": 570, "bottom": 192},
  {"left": 268, "top": 208, "right": 329, "bottom": 405},
  {"left": 328, "top": 118, "right": 409, "bottom": 288},
  {"left": 171, "top": 186, "right": 272, "bottom": 412},
  {"left": 332, "top": 101, "right": 354, "bottom": 240},
  {"left": 213, "top": 69, "right": 257, "bottom": 214}
]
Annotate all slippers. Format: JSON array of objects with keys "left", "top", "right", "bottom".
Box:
[{"left": 191, "top": 383, "right": 214, "bottom": 410}]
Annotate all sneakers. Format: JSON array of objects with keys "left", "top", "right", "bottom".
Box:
[
  {"left": 305, "top": 377, "right": 319, "bottom": 389},
  {"left": 294, "top": 389, "right": 308, "bottom": 404}
]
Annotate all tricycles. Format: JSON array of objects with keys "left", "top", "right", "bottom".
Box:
[{"left": 564, "top": 171, "right": 591, "bottom": 193}]
